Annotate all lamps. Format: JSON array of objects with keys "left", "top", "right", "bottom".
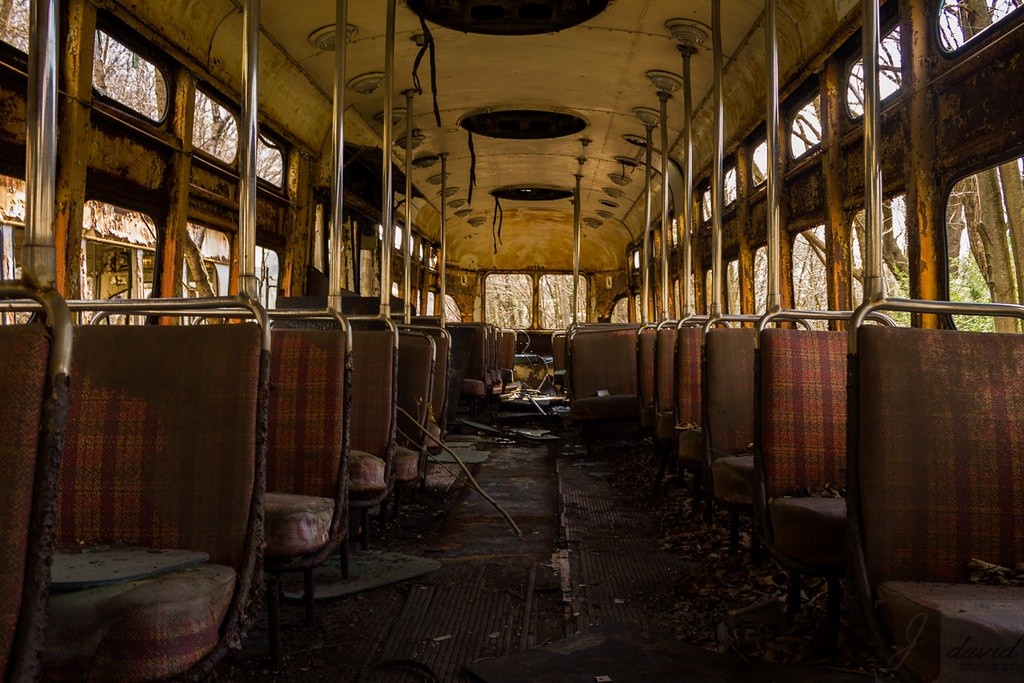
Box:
[
  {"left": 308, "top": 21, "right": 487, "bottom": 229},
  {"left": 583, "top": 14, "right": 715, "bottom": 238}
]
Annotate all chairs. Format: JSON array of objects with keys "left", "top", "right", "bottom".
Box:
[{"left": 0, "top": 270, "right": 1024, "bottom": 683}]
